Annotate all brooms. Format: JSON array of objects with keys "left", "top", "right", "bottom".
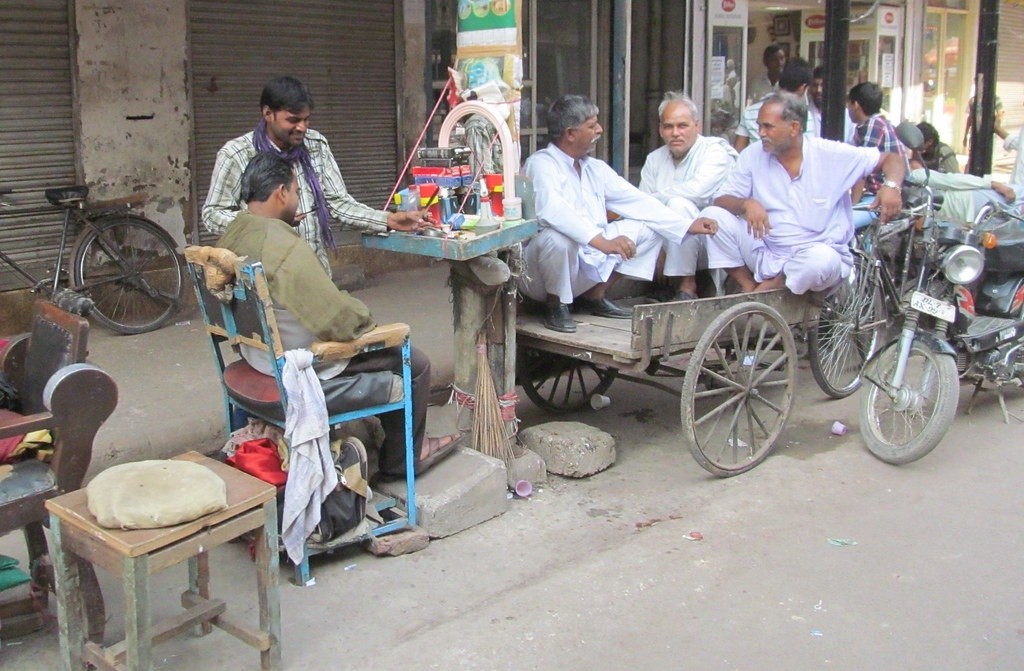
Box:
[{"left": 469, "top": 328, "right": 518, "bottom": 469}]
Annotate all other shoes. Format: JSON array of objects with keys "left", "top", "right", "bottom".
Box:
[
  {"left": 646, "top": 284, "right": 676, "bottom": 303},
  {"left": 669, "top": 289, "right": 697, "bottom": 304}
]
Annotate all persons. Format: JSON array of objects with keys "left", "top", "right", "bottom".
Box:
[
  {"left": 636, "top": 93, "right": 738, "bottom": 303},
  {"left": 201, "top": 77, "right": 436, "bottom": 283},
  {"left": 214, "top": 152, "right": 467, "bottom": 480},
  {"left": 843, "top": 83, "right": 910, "bottom": 230},
  {"left": 517, "top": 92, "right": 718, "bottom": 333},
  {"left": 909, "top": 122, "right": 960, "bottom": 173},
  {"left": 702, "top": 94, "right": 907, "bottom": 295},
  {"left": 993, "top": 104, "right": 1024, "bottom": 185},
  {"left": 726, "top": 59, "right": 737, "bottom": 103},
  {"left": 908, "top": 167, "right": 1024, "bottom": 225},
  {"left": 963, "top": 77, "right": 1005, "bottom": 176},
  {"left": 735, "top": 44, "right": 856, "bottom": 153}
]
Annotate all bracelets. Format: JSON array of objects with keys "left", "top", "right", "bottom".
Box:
[{"left": 883, "top": 181, "right": 902, "bottom": 193}]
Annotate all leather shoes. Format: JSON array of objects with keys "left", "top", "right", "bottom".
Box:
[
  {"left": 572, "top": 291, "right": 633, "bottom": 318},
  {"left": 542, "top": 301, "right": 577, "bottom": 333}
]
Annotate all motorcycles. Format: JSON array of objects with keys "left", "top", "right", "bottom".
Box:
[{"left": 857, "top": 120, "right": 1024, "bottom": 465}]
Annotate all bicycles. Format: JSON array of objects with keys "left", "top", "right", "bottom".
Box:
[{"left": 0, "top": 185, "right": 195, "bottom": 335}]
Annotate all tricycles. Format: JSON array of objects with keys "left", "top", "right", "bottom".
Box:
[{"left": 515, "top": 171, "right": 929, "bottom": 479}]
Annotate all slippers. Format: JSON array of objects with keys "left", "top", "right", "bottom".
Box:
[{"left": 401, "top": 430, "right": 468, "bottom": 476}]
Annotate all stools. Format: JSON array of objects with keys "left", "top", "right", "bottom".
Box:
[{"left": 44, "top": 450, "right": 280, "bottom": 671}]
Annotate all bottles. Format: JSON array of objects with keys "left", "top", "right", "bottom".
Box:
[
  {"left": 501, "top": 197, "right": 522, "bottom": 220},
  {"left": 406, "top": 184, "right": 420, "bottom": 213},
  {"left": 481, "top": 197, "right": 492, "bottom": 220}
]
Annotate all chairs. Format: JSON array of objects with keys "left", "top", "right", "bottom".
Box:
[
  {"left": 185, "top": 245, "right": 417, "bottom": 586},
  {"left": 0, "top": 299, "right": 119, "bottom": 671}
]
